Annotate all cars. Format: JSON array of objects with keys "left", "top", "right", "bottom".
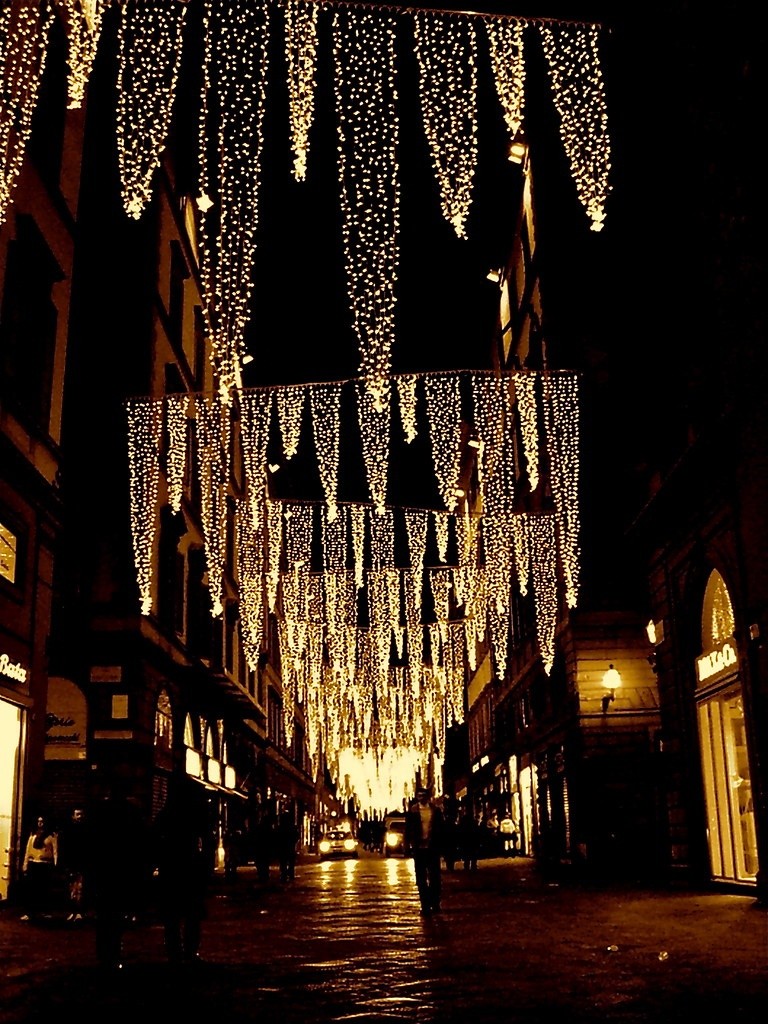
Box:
[
  {"left": 382, "top": 817, "right": 408, "bottom": 859},
  {"left": 319, "top": 830, "right": 360, "bottom": 859}
]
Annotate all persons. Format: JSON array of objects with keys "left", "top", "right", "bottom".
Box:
[
  {"left": 85, "top": 784, "right": 149, "bottom": 975},
  {"left": 20, "top": 810, "right": 84, "bottom": 923},
  {"left": 489, "top": 814, "right": 518, "bottom": 859},
  {"left": 358, "top": 820, "right": 387, "bottom": 855},
  {"left": 150, "top": 775, "right": 217, "bottom": 969},
  {"left": 252, "top": 813, "right": 299, "bottom": 882},
  {"left": 442, "top": 809, "right": 480, "bottom": 873},
  {"left": 405, "top": 788, "right": 446, "bottom": 917}
]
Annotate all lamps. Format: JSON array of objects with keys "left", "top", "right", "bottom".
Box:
[{"left": 601, "top": 664, "right": 621, "bottom": 713}]
